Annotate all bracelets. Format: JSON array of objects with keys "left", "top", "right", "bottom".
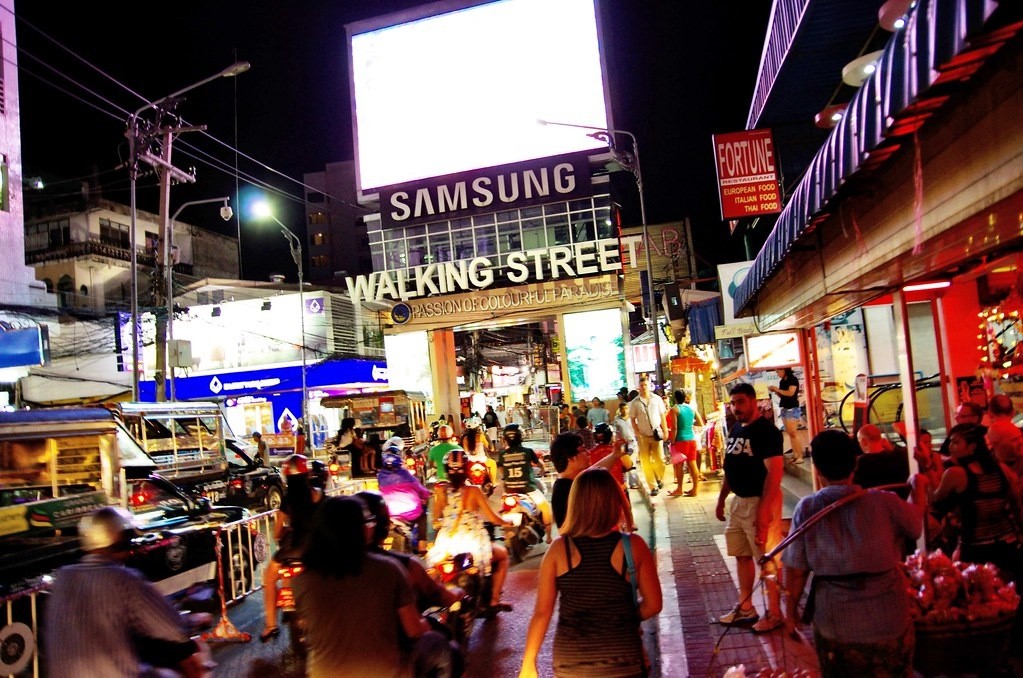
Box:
[{"left": 921, "top": 464, "right": 934, "bottom": 472}]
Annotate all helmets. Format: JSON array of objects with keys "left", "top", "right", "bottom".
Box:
[
  {"left": 465, "top": 418, "right": 479, "bottom": 429},
  {"left": 310, "top": 460, "right": 328, "bottom": 490},
  {"left": 353, "top": 492, "right": 390, "bottom": 539},
  {"left": 594, "top": 423, "right": 612, "bottom": 444},
  {"left": 381, "top": 447, "right": 403, "bottom": 468},
  {"left": 78, "top": 506, "right": 136, "bottom": 551},
  {"left": 437, "top": 425, "right": 454, "bottom": 438},
  {"left": 504, "top": 424, "right": 524, "bottom": 445},
  {"left": 442, "top": 450, "right": 468, "bottom": 473},
  {"left": 477, "top": 417, "right": 483, "bottom": 424},
  {"left": 282, "top": 453, "right": 312, "bottom": 476}
]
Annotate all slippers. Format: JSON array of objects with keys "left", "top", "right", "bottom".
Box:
[{"left": 491, "top": 603, "right": 512, "bottom": 612}]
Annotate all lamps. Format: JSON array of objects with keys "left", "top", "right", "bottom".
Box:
[
  {"left": 260, "top": 303, "right": 272, "bottom": 311},
  {"left": 211, "top": 307, "right": 221, "bottom": 317}
]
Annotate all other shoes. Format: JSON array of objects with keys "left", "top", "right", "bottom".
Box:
[
  {"left": 786, "top": 456, "right": 797, "bottom": 460},
  {"left": 651, "top": 488, "right": 659, "bottom": 495},
  {"left": 796, "top": 458, "right": 804, "bottom": 464},
  {"left": 658, "top": 481, "right": 663, "bottom": 489}
]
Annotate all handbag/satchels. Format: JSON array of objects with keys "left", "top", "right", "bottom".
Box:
[{"left": 653, "top": 427, "right": 664, "bottom": 441}]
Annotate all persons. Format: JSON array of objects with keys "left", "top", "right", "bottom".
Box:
[
  {"left": 297, "top": 426, "right": 306, "bottom": 455},
  {"left": 629, "top": 377, "right": 708, "bottom": 495},
  {"left": 988, "top": 394, "right": 1014, "bottom": 422},
  {"left": 260, "top": 455, "right": 326, "bottom": 645},
  {"left": 853, "top": 423, "right": 910, "bottom": 500},
  {"left": 439, "top": 404, "right": 501, "bottom": 453},
  {"left": 779, "top": 430, "right": 929, "bottom": 678},
  {"left": 40, "top": 506, "right": 212, "bottom": 678},
  {"left": 292, "top": 495, "right": 450, "bottom": 678},
  {"left": 380, "top": 424, "right": 514, "bottom": 613},
  {"left": 518, "top": 467, "right": 663, "bottom": 678},
  {"left": 337, "top": 418, "right": 378, "bottom": 478},
  {"left": 616, "top": 386, "right": 639, "bottom": 402},
  {"left": 552, "top": 396, "right": 639, "bottom": 489},
  {"left": 938, "top": 401, "right": 983, "bottom": 469},
  {"left": 495, "top": 401, "right": 549, "bottom": 442},
  {"left": 550, "top": 432, "right": 632, "bottom": 531},
  {"left": 717, "top": 383, "right": 786, "bottom": 633},
  {"left": 768, "top": 368, "right": 805, "bottom": 464},
  {"left": 912, "top": 423, "right": 1023, "bottom": 580},
  {"left": 253, "top": 432, "right": 269, "bottom": 460},
  {"left": 985, "top": 422, "right": 1023, "bottom": 506},
  {"left": 921, "top": 428, "right": 932, "bottom": 451},
  {"left": 352, "top": 490, "right": 466, "bottom": 678}
]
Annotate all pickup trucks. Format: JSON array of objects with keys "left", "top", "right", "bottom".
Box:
[{"left": 1, "top": 435, "right": 527, "bottom": 632}]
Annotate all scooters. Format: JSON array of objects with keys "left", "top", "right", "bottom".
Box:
[
  {"left": 424, "top": 552, "right": 482, "bottom": 678},
  {"left": 497, "top": 453, "right": 553, "bottom": 565},
  {"left": 271, "top": 507, "right": 309, "bottom": 660},
  {"left": 130, "top": 581, "right": 222, "bottom": 678}
]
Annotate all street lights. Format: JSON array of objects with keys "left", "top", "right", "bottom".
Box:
[
  {"left": 251, "top": 201, "right": 308, "bottom": 444},
  {"left": 538, "top": 120, "right": 665, "bottom": 398},
  {"left": 129, "top": 60, "right": 251, "bottom": 402}
]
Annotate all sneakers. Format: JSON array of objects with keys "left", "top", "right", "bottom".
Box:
[
  {"left": 753, "top": 612, "right": 785, "bottom": 634},
  {"left": 719, "top": 604, "right": 760, "bottom": 625}
]
